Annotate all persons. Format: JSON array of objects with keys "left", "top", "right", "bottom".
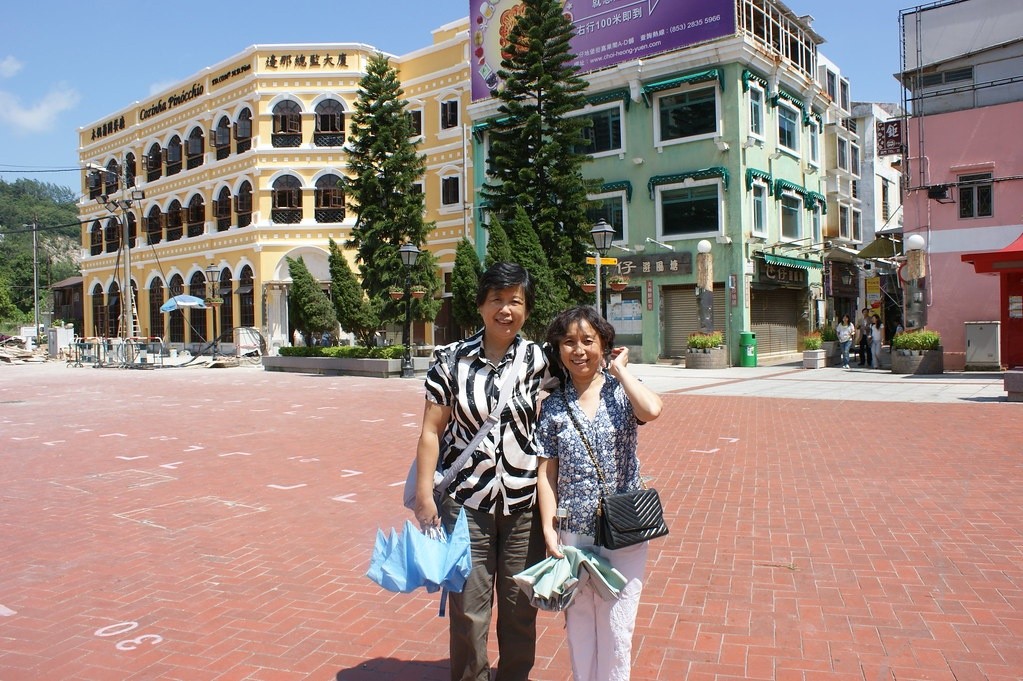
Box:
[
  {"left": 856, "top": 308, "right": 884, "bottom": 369},
  {"left": 836, "top": 314, "right": 855, "bottom": 369},
  {"left": 311, "top": 331, "right": 333, "bottom": 347},
  {"left": 414, "top": 261, "right": 561, "bottom": 681},
  {"left": 536, "top": 308, "right": 663, "bottom": 681}
]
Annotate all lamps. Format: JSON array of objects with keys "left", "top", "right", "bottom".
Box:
[
  {"left": 840, "top": 263, "right": 855, "bottom": 287},
  {"left": 633, "top": 158, "right": 645, "bottom": 165},
  {"left": 717, "top": 137, "right": 830, "bottom": 181}
]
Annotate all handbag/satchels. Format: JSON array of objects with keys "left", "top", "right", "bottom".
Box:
[
  {"left": 403, "top": 456, "right": 444, "bottom": 511},
  {"left": 594, "top": 488, "right": 670, "bottom": 550}
]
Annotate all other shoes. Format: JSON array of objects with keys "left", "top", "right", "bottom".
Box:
[
  {"left": 846, "top": 364, "right": 850, "bottom": 368},
  {"left": 868, "top": 362, "right": 871, "bottom": 366},
  {"left": 858, "top": 363, "right": 864, "bottom": 365}
]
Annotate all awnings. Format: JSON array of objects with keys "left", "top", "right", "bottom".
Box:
[
  {"left": 758, "top": 252, "right": 823, "bottom": 270},
  {"left": 857, "top": 238, "right": 902, "bottom": 258},
  {"left": 827, "top": 247, "right": 900, "bottom": 268}
]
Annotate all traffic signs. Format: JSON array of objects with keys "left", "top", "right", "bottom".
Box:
[{"left": 586, "top": 257, "right": 617, "bottom": 265}]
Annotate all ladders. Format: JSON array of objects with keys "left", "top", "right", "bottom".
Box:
[{"left": 117, "top": 286, "right": 142, "bottom": 337}]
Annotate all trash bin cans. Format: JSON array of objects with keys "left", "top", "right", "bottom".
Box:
[{"left": 739, "top": 332, "right": 757, "bottom": 367}]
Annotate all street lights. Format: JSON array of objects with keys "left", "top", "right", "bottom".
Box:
[
  {"left": 85, "top": 163, "right": 145, "bottom": 368},
  {"left": 398, "top": 241, "right": 421, "bottom": 378},
  {"left": 22, "top": 223, "right": 38, "bottom": 341},
  {"left": 589, "top": 218, "right": 617, "bottom": 320},
  {"left": 205, "top": 263, "right": 222, "bottom": 362}
]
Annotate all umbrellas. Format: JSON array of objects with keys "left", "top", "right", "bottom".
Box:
[
  {"left": 514, "top": 509, "right": 628, "bottom": 612},
  {"left": 366, "top": 479, "right": 472, "bottom": 617},
  {"left": 160, "top": 293, "right": 211, "bottom": 350}
]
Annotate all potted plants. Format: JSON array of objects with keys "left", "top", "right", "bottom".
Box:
[
  {"left": 685, "top": 330, "right": 727, "bottom": 369},
  {"left": 820, "top": 326, "right": 839, "bottom": 366},
  {"left": 608, "top": 276, "right": 628, "bottom": 291},
  {"left": 410, "top": 285, "right": 427, "bottom": 298},
  {"left": 803, "top": 325, "right": 828, "bottom": 369},
  {"left": 203, "top": 298, "right": 224, "bottom": 306},
  {"left": 389, "top": 286, "right": 404, "bottom": 301},
  {"left": 579, "top": 272, "right": 597, "bottom": 293},
  {"left": 891, "top": 329, "right": 944, "bottom": 375}
]
